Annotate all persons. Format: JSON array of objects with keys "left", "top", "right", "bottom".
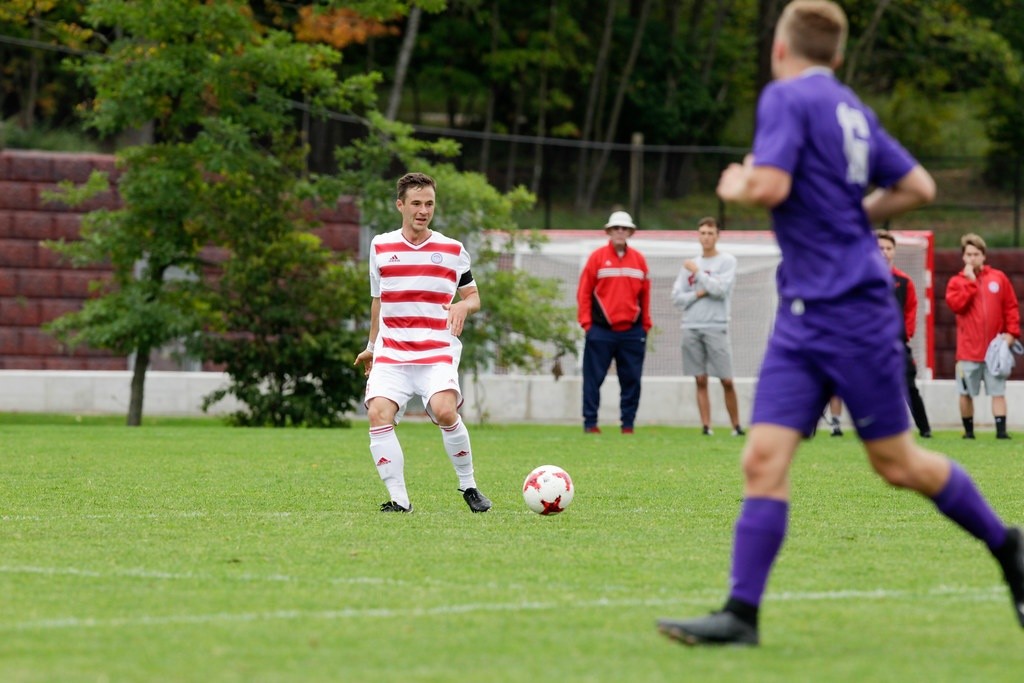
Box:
[
  {"left": 656, "top": 0, "right": 1024, "bottom": 649},
  {"left": 577, "top": 211, "right": 653, "bottom": 435},
  {"left": 873, "top": 230, "right": 933, "bottom": 438},
  {"left": 671, "top": 217, "right": 746, "bottom": 437},
  {"left": 945, "top": 232, "right": 1022, "bottom": 440},
  {"left": 353, "top": 173, "right": 490, "bottom": 512},
  {"left": 828, "top": 395, "right": 843, "bottom": 437}
]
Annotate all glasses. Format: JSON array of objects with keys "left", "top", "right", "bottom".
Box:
[{"left": 610, "top": 225, "right": 629, "bottom": 231}]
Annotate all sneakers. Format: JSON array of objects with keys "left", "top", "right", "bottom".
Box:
[
  {"left": 379, "top": 501, "right": 413, "bottom": 515},
  {"left": 462, "top": 488, "right": 492, "bottom": 513},
  {"left": 653, "top": 613, "right": 762, "bottom": 648},
  {"left": 996, "top": 525, "right": 1024, "bottom": 631}
]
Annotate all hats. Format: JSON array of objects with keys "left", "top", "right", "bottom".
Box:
[{"left": 604, "top": 211, "right": 637, "bottom": 238}]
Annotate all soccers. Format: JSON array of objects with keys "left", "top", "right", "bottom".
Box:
[{"left": 522, "top": 465, "right": 574, "bottom": 515}]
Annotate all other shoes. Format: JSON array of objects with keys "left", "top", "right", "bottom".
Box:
[
  {"left": 732, "top": 425, "right": 746, "bottom": 437},
  {"left": 995, "top": 432, "right": 1011, "bottom": 440},
  {"left": 962, "top": 433, "right": 975, "bottom": 439},
  {"left": 829, "top": 415, "right": 843, "bottom": 437},
  {"left": 621, "top": 427, "right": 633, "bottom": 434},
  {"left": 703, "top": 423, "right": 713, "bottom": 436},
  {"left": 922, "top": 431, "right": 932, "bottom": 438},
  {"left": 584, "top": 425, "right": 600, "bottom": 433}
]
